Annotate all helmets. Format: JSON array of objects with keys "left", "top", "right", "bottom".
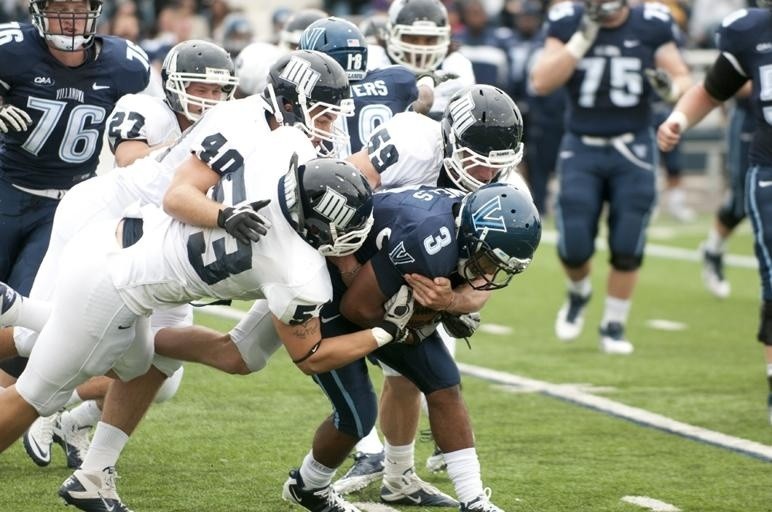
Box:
[{"left": 28, "top": 1, "right": 104, "bottom": 52}]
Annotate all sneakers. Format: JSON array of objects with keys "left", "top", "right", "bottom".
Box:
[
  {"left": 0, "top": 283, "right": 24, "bottom": 329},
  {"left": 555, "top": 289, "right": 596, "bottom": 341},
  {"left": 598, "top": 319, "right": 631, "bottom": 354},
  {"left": 694, "top": 241, "right": 733, "bottom": 297}
]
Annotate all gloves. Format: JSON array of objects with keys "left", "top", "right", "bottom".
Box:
[
  {"left": 584, "top": 0, "right": 624, "bottom": 25},
  {"left": 382, "top": 286, "right": 481, "bottom": 343},
  {"left": 647, "top": 67, "right": 685, "bottom": 105},
  {"left": 0, "top": 102, "right": 33, "bottom": 132}
]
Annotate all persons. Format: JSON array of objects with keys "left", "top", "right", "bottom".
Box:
[{"left": 5, "top": 1, "right": 772, "bottom": 511}]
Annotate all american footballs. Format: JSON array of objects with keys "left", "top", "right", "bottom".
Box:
[{"left": 405, "top": 299, "right": 438, "bottom": 330}]
